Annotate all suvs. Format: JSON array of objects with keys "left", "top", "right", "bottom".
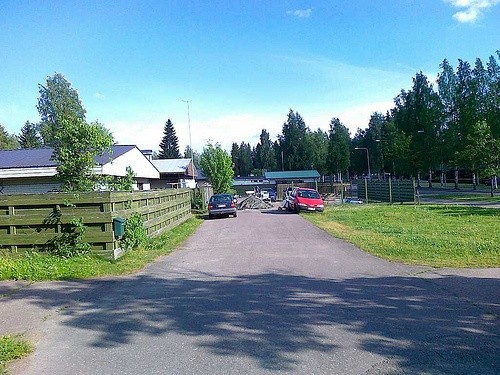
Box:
[{"left": 206, "top": 194, "right": 237, "bottom": 218}]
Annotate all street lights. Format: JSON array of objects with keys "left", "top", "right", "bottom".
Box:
[
  {"left": 418, "top": 130, "right": 447, "bottom": 187},
  {"left": 181, "top": 98, "right": 195, "bottom": 188},
  {"left": 376, "top": 139, "right": 395, "bottom": 176},
  {"left": 355, "top": 147, "right": 371, "bottom": 180}
]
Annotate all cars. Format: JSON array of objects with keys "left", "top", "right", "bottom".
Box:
[
  {"left": 288, "top": 187, "right": 325, "bottom": 213},
  {"left": 260, "top": 188, "right": 276, "bottom": 201}
]
